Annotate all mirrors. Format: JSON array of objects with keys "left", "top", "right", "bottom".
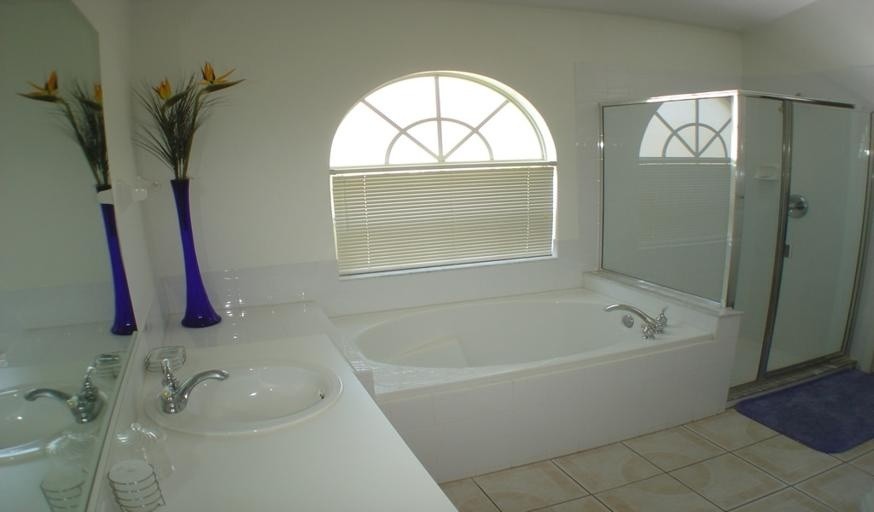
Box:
[{"left": 0, "top": 0, "right": 142, "bottom": 512}]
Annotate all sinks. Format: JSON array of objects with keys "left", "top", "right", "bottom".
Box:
[
  {"left": 0, "top": 382, "right": 82, "bottom": 461},
  {"left": 144, "top": 352, "right": 343, "bottom": 437}
]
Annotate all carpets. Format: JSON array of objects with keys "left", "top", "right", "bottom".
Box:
[{"left": 735, "top": 367, "right": 874, "bottom": 453}]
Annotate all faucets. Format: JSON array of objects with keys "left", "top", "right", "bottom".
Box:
[
  {"left": 154, "top": 357, "right": 230, "bottom": 415},
  {"left": 24, "top": 362, "right": 106, "bottom": 423},
  {"left": 604, "top": 302, "right": 668, "bottom": 339}
]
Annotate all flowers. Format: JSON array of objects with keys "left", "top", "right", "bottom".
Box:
[
  {"left": 14, "top": 70, "right": 109, "bottom": 186},
  {"left": 127, "top": 61, "right": 247, "bottom": 180}
]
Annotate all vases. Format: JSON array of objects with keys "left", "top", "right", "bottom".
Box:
[
  {"left": 96, "top": 185, "right": 138, "bottom": 335},
  {"left": 170, "top": 179, "right": 222, "bottom": 328}
]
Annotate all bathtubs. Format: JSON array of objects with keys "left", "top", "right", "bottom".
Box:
[{"left": 327, "top": 286, "right": 712, "bottom": 391}]
